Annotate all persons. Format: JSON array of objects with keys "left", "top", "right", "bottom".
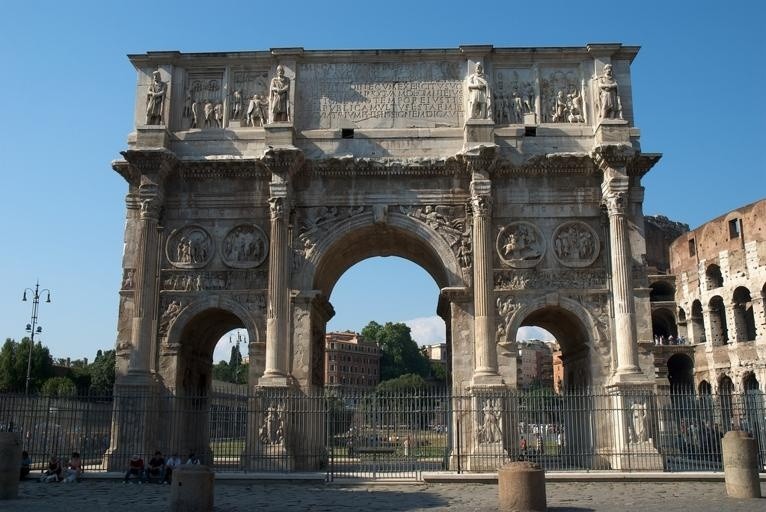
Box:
[
  {"left": 467, "top": 61, "right": 623, "bottom": 122},
  {"left": 518, "top": 422, "right": 564, "bottom": 458},
  {"left": 297, "top": 207, "right": 363, "bottom": 247},
  {"left": 163, "top": 453, "right": 181, "bottom": 484},
  {"left": 629, "top": 396, "right": 648, "bottom": 444},
  {"left": 225, "top": 227, "right": 263, "bottom": 262},
  {"left": 124, "top": 269, "right": 134, "bottom": 288},
  {"left": 163, "top": 273, "right": 226, "bottom": 290},
  {"left": 556, "top": 225, "right": 594, "bottom": 256},
  {"left": 20, "top": 451, "right": 31, "bottom": 479},
  {"left": 59, "top": 452, "right": 81, "bottom": 485},
  {"left": 493, "top": 271, "right": 604, "bottom": 289},
  {"left": 481, "top": 398, "right": 498, "bottom": 443},
  {"left": 501, "top": 223, "right": 539, "bottom": 260},
  {"left": 400, "top": 206, "right": 474, "bottom": 266},
  {"left": 263, "top": 401, "right": 278, "bottom": 445},
  {"left": 496, "top": 295, "right": 521, "bottom": 323},
  {"left": 40, "top": 455, "right": 61, "bottom": 483},
  {"left": 147, "top": 451, "right": 165, "bottom": 484},
  {"left": 654, "top": 332, "right": 685, "bottom": 346},
  {"left": 677, "top": 418, "right": 762, "bottom": 468},
  {"left": 403, "top": 437, "right": 411, "bottom": 461},
  {"left": 122, "top": 455, "right": 145, "bottom": 484},
  {"left": 177, "top": 237, "right": 209, "bottom": 263},
  {"left": 144, "top": 64, "right": 290, "bottom": 128},
  {"left": 186, "top": 452, "right": 200, "bottom": 465}
]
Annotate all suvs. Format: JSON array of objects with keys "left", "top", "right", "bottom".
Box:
[{"left": 33, "top": 423, "right": 66, "bottom": 445}]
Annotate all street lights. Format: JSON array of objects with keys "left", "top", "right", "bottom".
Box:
[
  {"left": 227, "top": 331, "right": 247, "bottom": 382},
  {"left": 21, "top": 278, "right": 52, "bottom": 395}
]
